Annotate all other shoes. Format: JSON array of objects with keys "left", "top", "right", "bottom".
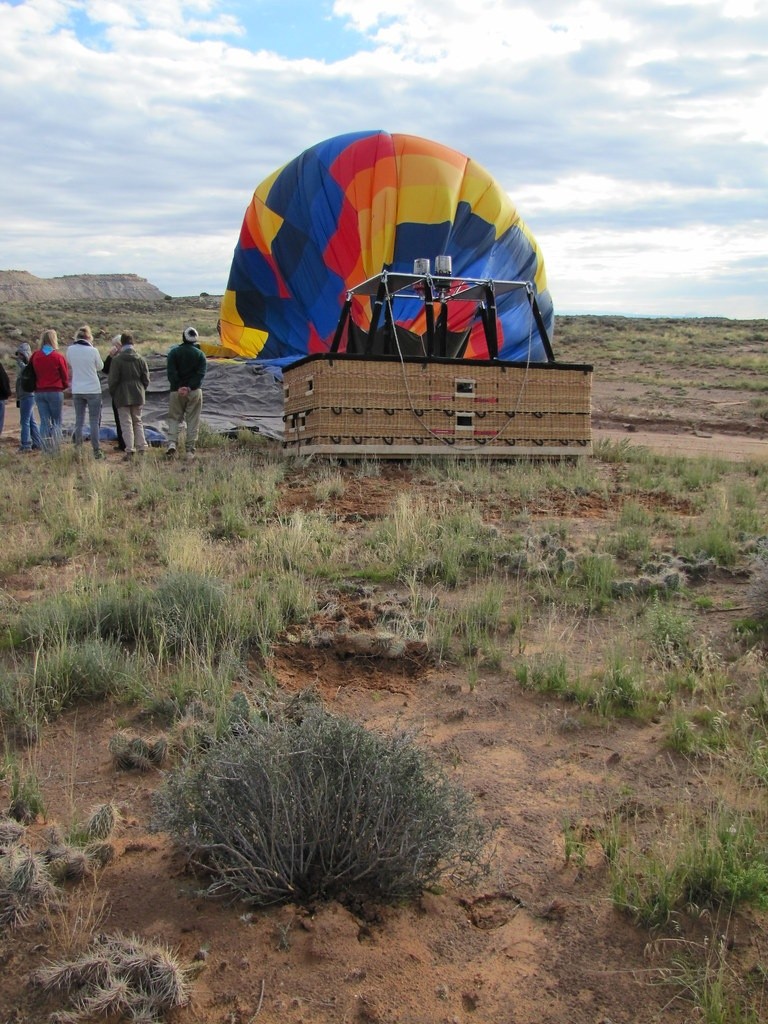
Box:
[
  {"left": 114, "top": 446, "right": 122, "bottom": 451},
  {"left": 166, "top": 444, "right": 176, "bottom": 454},
  {"left": 18, "top": 447, "right": 30, "bottom": 452},
  {"left": 138, "top": 450, "right": 144, "bottom": 455},
  {"left": 123, "top": 452, "right": 133, "bottom": 461},
  {"left": 186, "top": 451, "right": 194, "bottom": 459},
  {"left": 32, "top": 443, "right": 37, "bottom": 450},
  {"left": 94, "top": 450, "right": 102, "bottom": 459}
]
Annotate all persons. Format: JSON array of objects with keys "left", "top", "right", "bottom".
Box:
[
  {"left": 29, "top": 330, "right": 71, "bottom": 450},
  {"left": 101, "top": 331, "right": 150, "bottom": 461},
  {"left": 166, "top": 326, "right": 206, "bottom": 459},
  {"left": 9, "top": 342, "right": 42, "bottom": 452},
  {"left": 66, "top": 327, "right": 107, "bottom": 464},
  {"left": 0, "top": 362, "right": 12, "bottom": 434}
]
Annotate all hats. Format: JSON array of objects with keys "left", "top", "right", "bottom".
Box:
[{"left": 183, "top": 327, "right": 198, "bottom": 344}]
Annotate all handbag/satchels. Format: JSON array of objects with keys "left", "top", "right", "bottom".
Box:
[{"left": 20, "top": 363, "right": 36, "bottom": 392}]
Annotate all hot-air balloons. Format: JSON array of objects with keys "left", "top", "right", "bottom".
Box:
[{"left": 168, "top": 129, "right": 595, "bottom": 462}]
